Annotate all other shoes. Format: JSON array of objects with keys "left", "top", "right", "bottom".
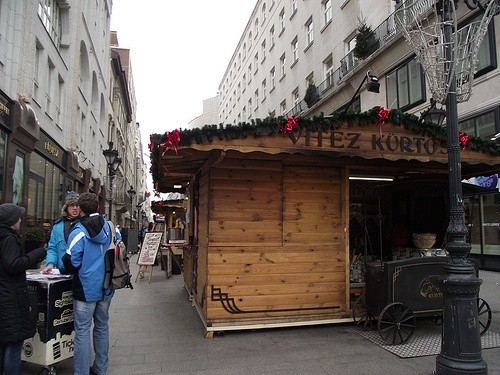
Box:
[{"left": 90, "top": 366, "right": 97, "bottom": 375}]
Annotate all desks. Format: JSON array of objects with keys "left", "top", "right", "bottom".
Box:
[{"left": 392, "top": 247, "right": 449, "bottom": 261}]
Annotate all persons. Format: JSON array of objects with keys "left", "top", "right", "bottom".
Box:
[
  {"left": 61, "top": 192, "right": 122, "bottom": 375},
  {"left": 0, "top": 202, "right": 48, "bottom": 375},
  {"left": 42, "top": 192, "right": 82, "bottom": 274}
]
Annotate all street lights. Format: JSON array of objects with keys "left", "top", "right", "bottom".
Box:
[
  {"left": 127, "top": 184, "right": 137, "bottom": 228},
  {"left": 135, "top": 202, "right": 144, "bottom": 233},
  {"left": 101, "top": 141, "right": 123, "bottom": 220}
]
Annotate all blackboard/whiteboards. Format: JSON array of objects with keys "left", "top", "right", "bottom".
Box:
[{"left": 137, "top": 232, "right": 163, "bottom": 265}]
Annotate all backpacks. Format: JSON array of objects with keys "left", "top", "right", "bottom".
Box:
[{"left": 104, "top": 221, "right": 131, "bottom": 289}]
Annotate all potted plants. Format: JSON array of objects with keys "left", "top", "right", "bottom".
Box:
[{"left": 25, "top": 227, "right": 45, "bottom": 255}]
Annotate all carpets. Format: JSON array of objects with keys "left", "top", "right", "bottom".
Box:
[{"left": 351, "top": 319, "right": 500, "bottom": 359}]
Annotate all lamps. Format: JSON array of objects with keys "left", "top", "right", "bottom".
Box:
[
  {"left": 348, "top": 175, "right": 395, "bottom": 181},
  {"left": 344, "top": 70, "right": 380, "bottom": 113}
]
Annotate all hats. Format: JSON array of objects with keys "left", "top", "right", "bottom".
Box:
[
  {"left": 0, "top": 203, "right": 25, "bottom": 226},
  {"left": 61, "top": 192, "right": 81, "bottom": 216}
]
